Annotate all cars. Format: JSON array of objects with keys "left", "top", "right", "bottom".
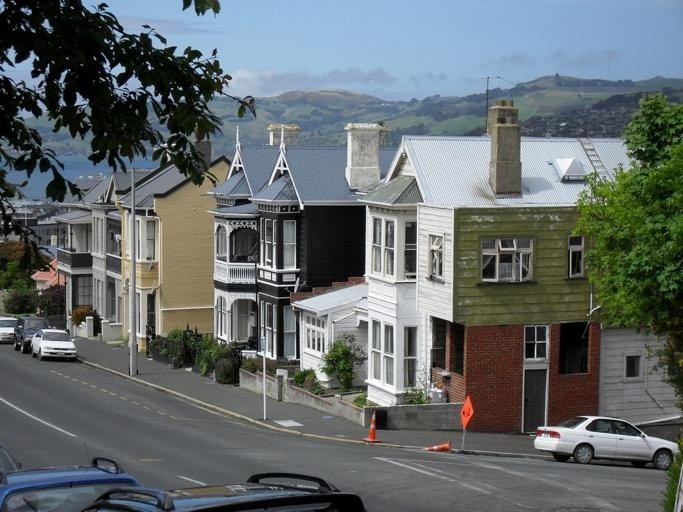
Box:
[
  {"left": 0, "top": 315, "right": 18, "bottom": 345},
  {"left": 29, "top": 328, "right": 78, "bottom": 363},
  {"left": 532, "top": 414, "right": 682, "bottom": 472}
]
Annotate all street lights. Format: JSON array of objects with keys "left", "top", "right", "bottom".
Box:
[{"left": 259, "top": 335, "right": 267, "bottom": 423}]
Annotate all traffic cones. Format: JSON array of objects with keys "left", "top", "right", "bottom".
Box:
[
  {"left": 418, "top": 439, "right": 453, "bottom": 453},
  {"left": 363, "top": 409, "right": 382, "bottom": 444}
]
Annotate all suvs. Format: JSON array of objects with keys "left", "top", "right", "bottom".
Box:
[
  {"left": 76, "top": 471, "right": 369, "bottom": 512},
  {"left": 0, "top": 454, "right": 146, "bottom": 512},
  {"left": 12, "top": 314, "right": 52, "bottom": 355}
]
